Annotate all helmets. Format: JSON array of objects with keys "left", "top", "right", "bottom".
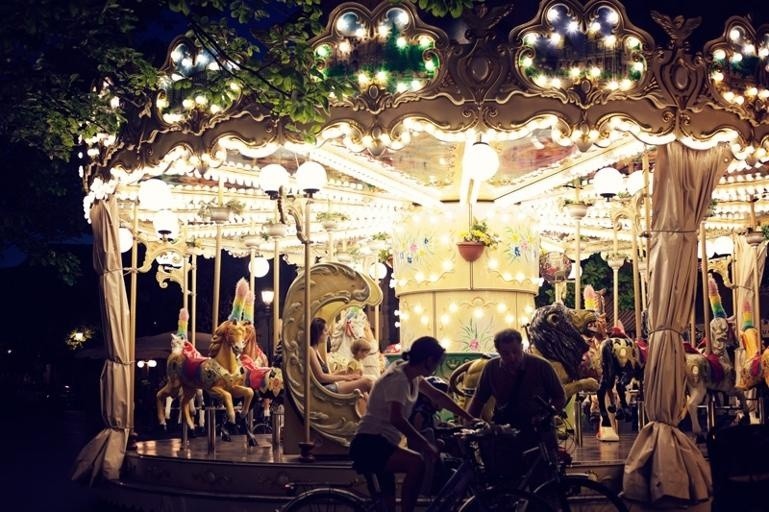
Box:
[{"left": 425, "top": 375, "right": 448, "bottom": 392}]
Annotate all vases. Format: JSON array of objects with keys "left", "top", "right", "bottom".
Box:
[{"left": 458, "top": 241, "right": 485, "bottom": 262}]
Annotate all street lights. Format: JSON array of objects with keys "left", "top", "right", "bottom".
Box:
[
  {"left": 261, "top": 287, "right": 275, "bottom": 366},
  {"left": 137, "top": 359, "right": 157, "bottom": 378},
  {"left": 76, "top": 327, "right": 85, "bottom": 343}
]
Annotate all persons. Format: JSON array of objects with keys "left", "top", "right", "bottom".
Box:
[
  {"left": 349, "top": 336, "right": 492, "bottom": 512},
  {"left": 309, "top": 317, "right": 373, "bottom": 394},
  {"left": 346, "top": 339, "right": 372, "bottom": 378},
  {"left": 464, "top": 329, "right": 566, "bottom": 511},
  {"left": 406, "top": 376, "right": 470, "bottom": 498}
]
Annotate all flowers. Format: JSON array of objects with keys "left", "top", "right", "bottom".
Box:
[{"left": 461, "top": 220, "right": 503, "bottom": 246}]
